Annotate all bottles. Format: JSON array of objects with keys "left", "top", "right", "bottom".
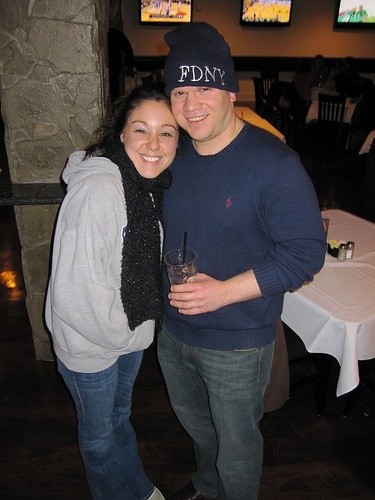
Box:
[{"left": 337, "top": 241, "right": 354, "bottom": 261}]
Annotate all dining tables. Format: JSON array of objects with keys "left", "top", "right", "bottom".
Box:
[
  {"left": 307, "top": 119, "right": 361, "bottom": 170},
  {"left": 291, "top": 210, "right": 375, "bottom": 425}
]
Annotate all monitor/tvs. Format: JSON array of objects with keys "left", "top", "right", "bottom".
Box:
[
  {"left": 138, "top": 0, "right": 193, "bottom": 25},
  {"left": 240, "top": 0, "right": 293, "bottom": 27},
  {"left": 335, "top": 0, "right": 375, "bottom": 29}
]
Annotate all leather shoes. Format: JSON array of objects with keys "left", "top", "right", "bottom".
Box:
[{"left": 169, "top": 481, "right": 209, "bottom": 500}]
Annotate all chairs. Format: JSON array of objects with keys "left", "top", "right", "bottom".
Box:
[
  {"left": 318, "top": 93, "right": 347, "bottom": 124},
  {"left": 252, "top": 68, "right": 286, "bottom": 122}
]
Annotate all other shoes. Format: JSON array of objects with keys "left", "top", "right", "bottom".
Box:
[{"left": 147, "top": 487, "right": 166, "bottom": 500}]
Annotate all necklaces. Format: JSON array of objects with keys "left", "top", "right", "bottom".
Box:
[{"left": 232, "top": 119, "right": 242, "bottom": 139}]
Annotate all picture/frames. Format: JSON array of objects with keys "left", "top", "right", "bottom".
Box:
[
  {"left": 332, "top": 0, "right": 375, "bottom": 31},
  {"left": 239, "top": 0, "right": 292, "bottom": 27},
  {"left": 138, "top": 0, "right": 194, "bottom": 26}
]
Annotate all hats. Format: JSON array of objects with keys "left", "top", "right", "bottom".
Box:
[{"left": 163, "top": 21, "right": 240, "bottom": 97}]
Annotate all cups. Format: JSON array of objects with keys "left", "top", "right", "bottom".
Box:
[{"left": 164, "top": 249, "right": 198, "bottom": 286}]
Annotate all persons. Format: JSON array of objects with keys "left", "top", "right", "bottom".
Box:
[
  {"left": 44, "top": 82, "right": 181, "bottom": 500},
  {"left": 107, "top": 27, "right": 136, "bottom": 102},
  {"left": 278, "top": 54, "right": 375, "bottom": 182},
  {"left": 141, "top": 0, "right": 191, "bottom": 22},
  {"left": 338, "top": 5, "right": 368, "bottom": 23},
  {"left": 161, "top": 21, "right": 327, "bottom": 500}
]
